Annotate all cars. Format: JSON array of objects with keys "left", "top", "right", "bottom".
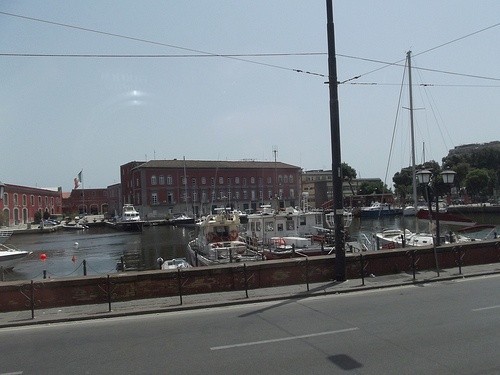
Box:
[{"left": 43, "top": 219, "right": 61, "bottom": 227}]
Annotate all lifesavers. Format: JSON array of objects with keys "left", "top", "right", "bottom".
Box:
[{"left": 230, "top": 230, "right": 238, "bottom": 240}]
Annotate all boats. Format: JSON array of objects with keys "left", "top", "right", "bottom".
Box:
[
  {"left": 64, "top": 223, "right": 90, "bottom": 230},
  {"left": 187, "top": 205, "right": 268, "bottom": 269},
  {"left": 161, "top": 259, "right": 191, "bottom": 270},
  {"left": 0, "top": 244, "right": 31, "bottom": 271},
  {"left": 376, "top": 224, "right": 481, "bottom": 247},
  {"left": 240, "top": 204, "right": 369, "bottom": 257},
  {"left": 360, "top": 200, "right": 448, "bottom": 218}
]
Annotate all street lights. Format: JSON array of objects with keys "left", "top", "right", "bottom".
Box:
[{"left": 416, "top": 163, "right": 456, "bottom": 278}]
